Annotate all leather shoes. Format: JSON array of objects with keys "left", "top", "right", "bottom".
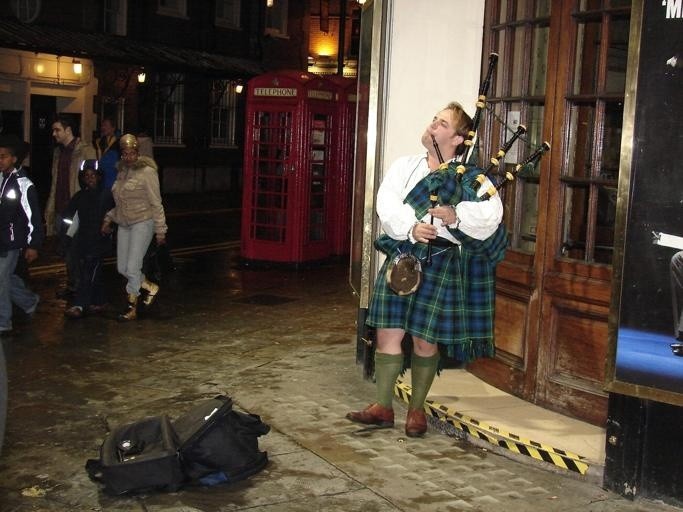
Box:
[
  {"left": 403, "top": 406, "right": 427, "bottom": 437},
  {"left": 342, "top": 402, "right": 396, "bottom": 429}
]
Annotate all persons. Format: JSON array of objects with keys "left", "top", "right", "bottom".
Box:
[
  {"left": 0, "top": 142, "right": 40, "bottom": 337},
  {"left": 41, "top": 111, "right": 169, "bottom": 321},
  {"left": 342, "top": 101, "right": 505, "bottom": 438}
]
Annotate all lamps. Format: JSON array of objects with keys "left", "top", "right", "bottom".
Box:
[
  {"left": 234, "top": 81, "right": 245, "bottom": 94},
  {"left": 137, "top": 68, "right": 146, "bottom": 83}
]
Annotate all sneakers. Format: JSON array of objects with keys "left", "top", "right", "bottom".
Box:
[{"left": 52, "top": 289, "right": 110, "bottom": 318}]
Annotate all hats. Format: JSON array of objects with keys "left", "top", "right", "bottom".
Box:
[{"left": 118, "top": 134, "right": 141, "bottom": 151}]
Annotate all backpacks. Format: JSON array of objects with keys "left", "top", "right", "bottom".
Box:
[{"left": 84, "top": 393, "right": 276, "bottom": 497}]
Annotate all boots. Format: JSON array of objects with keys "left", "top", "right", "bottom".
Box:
[
  {"left": 139, "top": 276, "right": 161, "bottom": 306},
  {"left": 116, "top": 293, "right": 138, "bottom": 321}
]
